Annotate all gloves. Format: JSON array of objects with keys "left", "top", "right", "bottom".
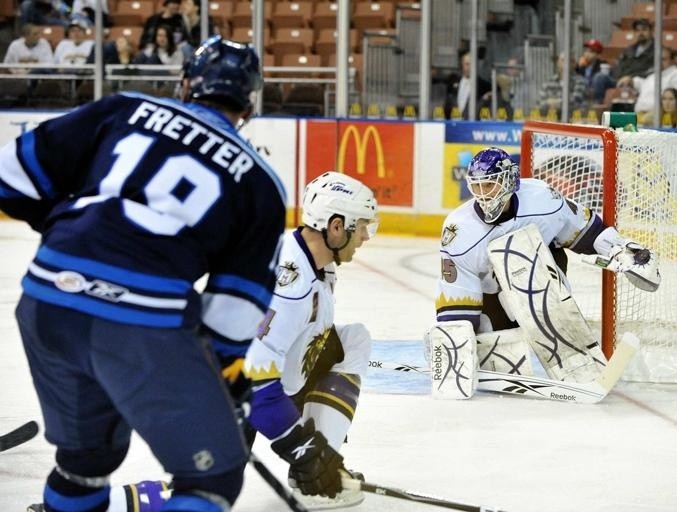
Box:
[{"left": 271, "top": 417, "right": 345, "bottom": 499}]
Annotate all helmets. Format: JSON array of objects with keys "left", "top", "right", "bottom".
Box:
[
  {"left": 176, "top": 33, "right": 266, "bottom": 134},
  {"left": 296, "top": 168, "right": 379, "bottom": 270},
  {"left": 457, "top": 145, "right": 524, "bottom": 228}
]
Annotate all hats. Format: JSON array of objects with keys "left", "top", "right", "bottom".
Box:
[{"left": 583, "top": 39, "right": 604, "bottom": 54}]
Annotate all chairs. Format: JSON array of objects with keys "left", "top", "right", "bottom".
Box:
[
  {"left": 200, "top": 2, "right": 232, "bottom": 24},
  {"left": 281, "top": 52, "right": 327, "bottom": 113},
  {"left": 85, "top": 28, "right": 95, "bottom": 39},
  {"left": 109, "top": 0, "right": 152, "bottom": 25},
  {"left": 234, "top": 0, "right": 273, "bottom": 27},
  {"left": 594, "top": 87, "right": 639, "bottom": 118},
  {"left": 661, "top": 1, "right": 676, "bottom": 28},
  {"left": 263, "top": 54, "right": 282, "bottom": 107},
  {"left": 315, "top": 1, "right": 348, "bottom": 27},
  {"left": 35, "top": 23, "right": 62, "bottom": 43},
  {"left": 619, "top": 1, "right": 657, "bottom": 29},
  {"left": 317, "top": 28, "right": 361, "bottom": 53},
  {"left": 351, "top": 2, "right": 391, "bottom": 27},
  {"left": 107, "top": 27, "right": 142, "bottom": 47},
  {"left": 231, "top": 27, "right": 271, "bottom": 47},
  {"left": 155, "top": 0, "right": 165, "bottom": 12},
  {"left": 359, "top": 28, "right": 397, "bottom": 52},
  {"left": 269, "top": 28, "right": 314, "bottom": 50},
  {"left": 399, "top": 0, "right": 423, "bottom": 23},
  {"left": 272, "top": 0, "right": 313, "bottom": 26},
  {"left": 602, "top": 29, "right": 633, "bottom": 59},
  {"left": 658, "top": 31, "right": 676, "bottom": 51},
  {"left": 328, "top": 54, "right": 362, "bottom": 103}
]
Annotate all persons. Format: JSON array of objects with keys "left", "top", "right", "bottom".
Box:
[
  {"left": 534, "top": 49, "right": 589, "bottom": 120},
  {"left": 68, "top": 1, "right": 117, "bottom": 29},
  {"left": 107, "top": 29, "right": 135, "bottom": 63},
  {"left": 634, "top": 44, "right": 677, "bottom": 114},
  {"left": 55, "top": 22, "right": 96, "bottom": 71},
  {"left": 638, "top": 88, "right": 677, "bottom": 134},
  {"left": 575, "top": 37, "right": 611, "bottom": 87},
  {"left": 589, "top": 17, "right": 655, "bottom": 106},
  {"left": 15, "top": 0, "right": 72, "bottom": 31},
  {"left": 181, "top": 0, "right": 214, "bottom": 48},
  {"left": 85, "top": 28, "right": 122, "bottom": 66},
  {"left": 3, "top": 22, "right": 54, "bottom": 73},
  {"left": 0, "top": 35, "right": 288, "bottom": 512},
  {"left": 28, "top": 169, "right": 379, "bottom": 510},
  {"left": 433, "top": 152, "right": 659, "bottom": 404},
  {"left": 133, "top": 24, "right": 195, "bottom": 66},
  {"left": 139, "top": 0, "right": 187, "bottom": 50},
  {"left": 443, "top": 48, "right": 501, "bottom": 122}
]
